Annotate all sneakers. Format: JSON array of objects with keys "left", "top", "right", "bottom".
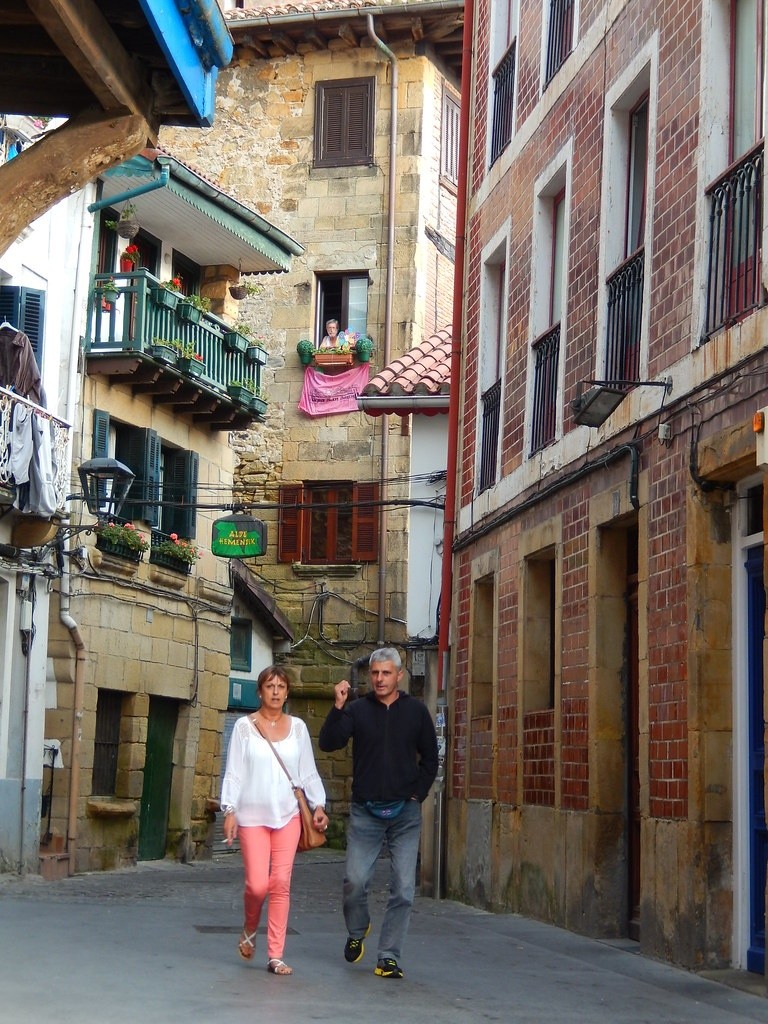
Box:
[
  {"left": 375, "top": 958, "right": 403, "bottom": 979},
  {"left": 345, "top": 923, "right": 372, "bottom": 962}
]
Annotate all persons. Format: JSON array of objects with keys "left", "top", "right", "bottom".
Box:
[
  {"left": 219, "top": 666, "right": 328, "bottom": 973},
  {"left": 319, "top": 319, "right": 340, "bottom": 348},
  {"left": 319, "top": 647, "right": 438, "bottom": 979}
]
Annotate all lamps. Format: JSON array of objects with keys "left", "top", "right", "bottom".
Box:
[
  {"left": 29, "top": 457, "right": 136, "bottom": 562},
  {"left": 568, "top": 375, "right": 673, "bottom": 427}
]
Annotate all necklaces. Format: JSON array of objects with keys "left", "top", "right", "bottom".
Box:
[{"left": 259, "top": 710, "right": 282, "bottom": 727}]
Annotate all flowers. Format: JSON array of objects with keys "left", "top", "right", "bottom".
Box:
[
  {"left": 249, "top": 336, "right": 266, "bottom": 348},
  {"left": 95, "top": 521, "right": 204, "bottom": 566},
  {"left": 121, "top": 245, "right": 140, "bottom": 264},
  {"left": 159, "top": 273, "right": 183, "bottom": 293},
  {"left": 101, "top": 276, "right": 121, "bottom": 293}
]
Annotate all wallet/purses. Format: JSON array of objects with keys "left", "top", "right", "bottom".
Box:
[{"left": 364, "top": 801, "right": 406, "bottom": 820}]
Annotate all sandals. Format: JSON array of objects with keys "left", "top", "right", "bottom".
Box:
[
  {"left": 267, "top": 959, "right": 293, "bottom": 975},
  {"left": 238, "top": 931, "right": 257, "bottom": 961}
]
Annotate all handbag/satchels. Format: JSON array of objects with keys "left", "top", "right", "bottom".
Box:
[
  {"left": 212, "top": 511, "right": 267, "bottom": 558},
  {"left": 294, "top": 787, "right": 327, "bottom": 852}
]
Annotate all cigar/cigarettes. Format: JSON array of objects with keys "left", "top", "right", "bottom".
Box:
[{"left": 221, "top": 839, "right": 228, "bottom": 843}]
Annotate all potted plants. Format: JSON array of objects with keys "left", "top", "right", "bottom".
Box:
[
  {"left": 151, "top": 337, "right": 185, "bottom": 364},
  {"left": 227, "top": 377, "right": 271, "bottom": 414},
  {"left": 224, "top": 323, "right": 252, "bottom": 353},
  {"left": 297, "top": 336, "right": 374, "bottom": 367},
  {"left": 177, "top": 295, "right": 212, "bottom": 324},
  {"left": 104, "top": 205, "right": 140, "bottom": 238},
  {"left": 228, "top": 282, "right": 261, "bottom": 299}
]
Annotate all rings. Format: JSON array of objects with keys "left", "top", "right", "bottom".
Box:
[{"left": 323, "top": 825, "right": 327, "bottom": 830}]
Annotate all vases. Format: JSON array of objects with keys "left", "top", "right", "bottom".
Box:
[
  {"left": 150, "top": 287, "right": 179, "bottom": 310},
  {"left": 104, "top": 289, "right": 118, "bottom": 302},
  {"left": 247, "top": 346, "right": 269, "bottom": 364},
  {"left": 120, "top": 258, "right": 133, "bottom": 272}
]
